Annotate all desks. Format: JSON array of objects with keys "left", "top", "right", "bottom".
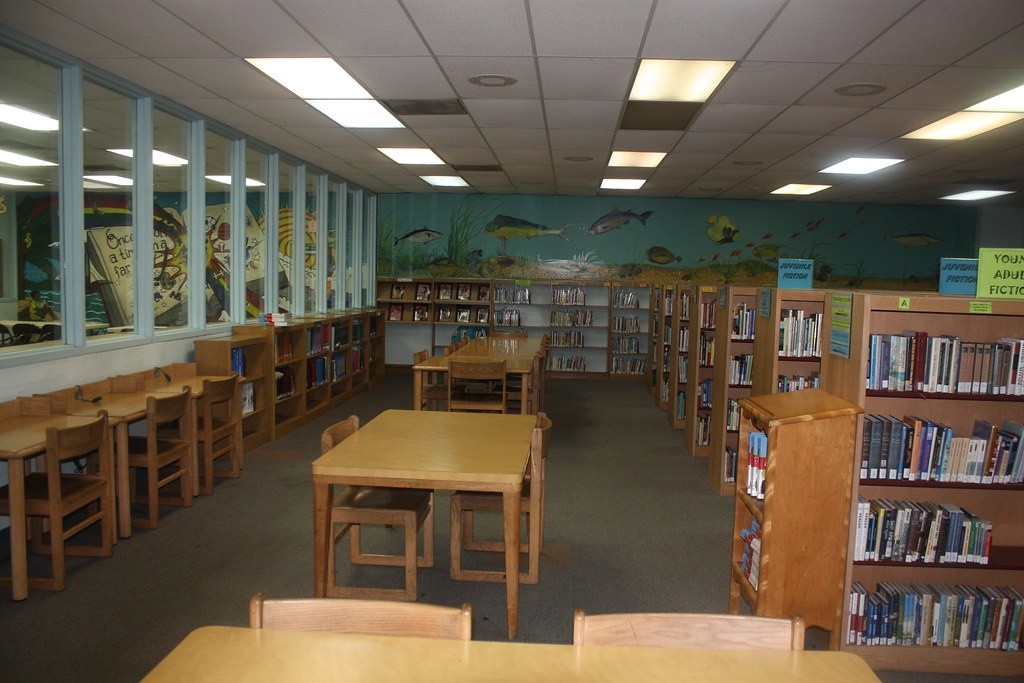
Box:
[
  {"left": 1, "top": 362, "right": 247, "bottom": 602},
  {"left": 0, "top": 320, "right": 109, "bottom": 330},
  {"left": 312, "top": 408, "right": 537, "bottom": 641},
  {"left": 412, "top": 358, "right": 534, "bottom": 414},
  {"left": 450, "top": 337, "right": 543, "bottom": 357},
  {"left": 139, "top": 625, "right": 884, "bottom": 683}
]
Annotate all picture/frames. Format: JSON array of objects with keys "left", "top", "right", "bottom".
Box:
[{"left": 387, "top": 283, "right": 489, "bottom": 324}]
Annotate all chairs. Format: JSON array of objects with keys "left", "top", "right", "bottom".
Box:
[
  {"left": 86, "top": 385, "right": 192, "bottom": 528},
  {"left": 248, "top": 591, "right": 472, "bottom": 641},
  {"left": 413, "top": 332, "right": 550, "bottom": 413},
  {"left": 161, "top": 370, "right": 240, "bottom": 496},
  {"left": 0, "top": 323, "right": 65, "bottom": 347},
  {"left": 0, "top": 410, "right": 114, "bottom": 590},
  {"left": 450, "top": 411, "right": 553, "bottom": 585},
  {"left": 321, "top": 414, "right": 434, "bottom": 601},
  {"left": 573, "top": 609, "right": 807, "bottom": 653}
]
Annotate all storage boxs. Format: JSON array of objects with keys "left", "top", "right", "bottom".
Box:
[{"left": 241, "top": 382, "right": 254, "bottom": 414}]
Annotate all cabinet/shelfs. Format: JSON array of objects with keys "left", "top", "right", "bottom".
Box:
[
  {"left": 649, "top": 282, "right": 1023, "bottom": 677},
  {"left": 194, "top": 335, "right": 272, "bottom": 462},
  {"left": 375, "top": 275, "right": 493, "bottom": 374},
  {"left": 611, "top": 281, "right": 653, "bottom": 382},
  {"left": 231, "top": 308, "right": 385, "bottom": 442},
  {"left": 492, "top": 279, "right": 610, "bottom": 381}
]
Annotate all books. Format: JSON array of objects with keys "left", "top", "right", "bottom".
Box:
[
  {"left": 846, "top": 580, "right": 1024, "bottom": 653},
  {"left": 663, "top": 292, "right": 672, "bottom": 405},
  {"left": 730, "top": 302, "right": 754, "bottom": 340},
  {"left": 493, "top": 309, "right": 520, "bottom": 327},
  {"left": 779, "top": 307, "right": 823, "bottom": 393},
  {"left": 728, "top": 353, "right": 754, "bottom": 387},
  {"left": 699, "top": 301, "right": 717, "bottom": 367},
  {"left": 696, "top": 378, "right": 712, "bottom": 446},
  {"left": 551, "top": 354, "right": 586, "bottom": 371},
  {"left": 611, "top": 356, "right": 648, "bottom": 374},
  {"left": 613, "top": 316, "right": 640, "bottom": 355},
  {"left": 725, "top": 444, "right": 737, "bottom": 483},
  {"left": 551, "top": 330, "right": 583, "bottom": 347},
  {"left": 740, "top": 515, "right": 761, "bottom": 592},
  {"left": 853, "top": 495, "right": 992, "bottom": 565},
  {"left": 865, "top": 331, "right": 1024, "bottom": 394},
  {"left": 388, "top": 283, "right": 491, "bottom": 324},
  {"left": 727, "top": 398, "right": 741, "bottom": 431},
  {"left": 553, "top": 288, "right": 585, "bottom": 305},
  {"left": 496, "top": 287, "right": 531, "bottom": 303},
  {"left": 613, "top": 292, "right": 639, "bottom": 309},
  {"left": 677, "top": 293, "right": 690, "bottom": 420},
  {"left": 745, "top": 430, "right": 767, "bottom": 500},
  {"left": 231, "top": 313, "right": 377, "bottom": 416},
  {"left": 551, "top": 309, "right": 592, "bottom": 326},
  {"left": 860, "top": 414, "right": 1024, "bottom": 484}
]
